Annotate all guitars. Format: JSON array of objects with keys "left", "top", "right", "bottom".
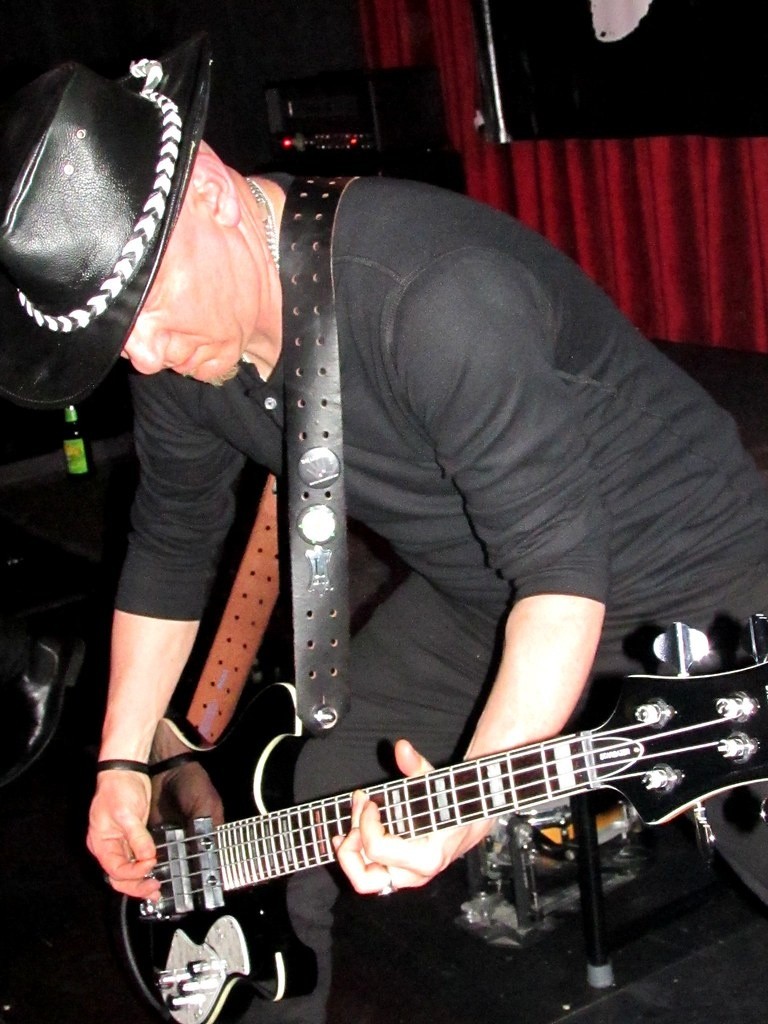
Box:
[{"left": 114, "top": 611, "right": 767, "bottom": 1024}]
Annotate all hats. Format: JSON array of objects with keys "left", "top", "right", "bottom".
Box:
[{"left": 0, "top": 21, "right": 212, "bottom": 409}]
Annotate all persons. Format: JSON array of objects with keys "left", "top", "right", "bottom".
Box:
[
  {"left": 0, "top": 373, "right": 246, "bottom": 1024},
  {"left": 0, "top": 53, "right": 767, "bottom": 1024}
]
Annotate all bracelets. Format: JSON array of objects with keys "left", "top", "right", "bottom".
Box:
[
  {"left": 149, "top": 749, "right": 201, "bottom": 775},
  {"left": 95, "top": 757, "right": 152, "bottom": 776}
]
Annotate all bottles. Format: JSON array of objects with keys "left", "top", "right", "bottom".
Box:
[{"left": 60, "top": 402, "right": 97, "bottom": 486}]
